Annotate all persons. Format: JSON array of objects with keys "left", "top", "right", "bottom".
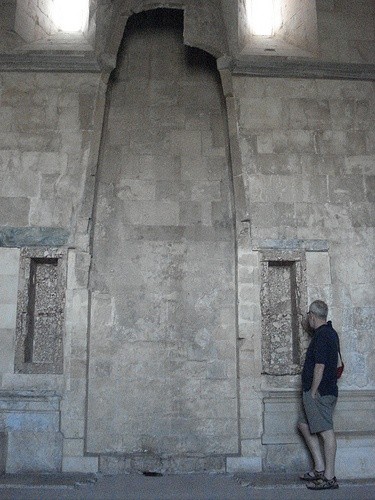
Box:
[{"left": 298, "top": 300, "right": 340, "bottom": 490}]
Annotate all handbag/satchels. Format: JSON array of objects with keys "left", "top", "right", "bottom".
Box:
[{"left": 337, "top": 366, "right": 344, "bottom": 377}]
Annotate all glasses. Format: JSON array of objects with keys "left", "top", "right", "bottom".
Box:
[{"left": 307, "top": 311, "right": 313, "bottom": 315}]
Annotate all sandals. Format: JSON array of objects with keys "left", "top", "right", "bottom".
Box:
[
  {"left": 299, "top": 468, "right": 325, "bottom": 480},
  {"left": 306, "top": 476, "right": 339, "bottom": 490}
]
